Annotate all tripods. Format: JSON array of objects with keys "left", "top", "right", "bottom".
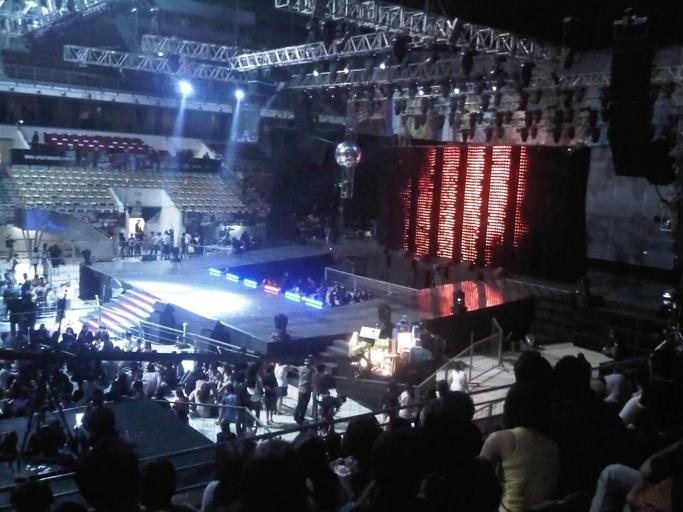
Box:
[{"left": 17, "top": 369, "right": 74, "bottom": 465}]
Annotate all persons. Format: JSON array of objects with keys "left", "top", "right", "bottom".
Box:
[
  {"left": 32, "top": 131, "right": 39, "bottom": 142},
  {"left": 203, "top": 152, "right": 209, "bottom": 159},
  {"left": 1, "top": 219, "right": 683, "bottom": 512}
]
